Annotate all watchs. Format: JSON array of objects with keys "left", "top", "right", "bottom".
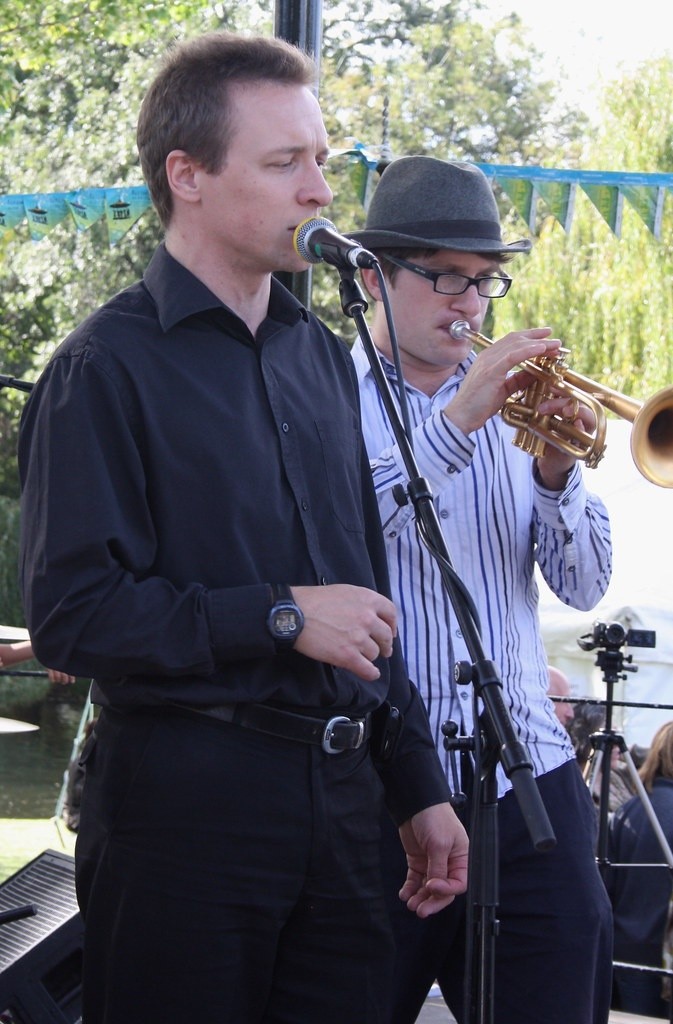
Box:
[{"left": 268, "top": 582, "right": 304, "bottom": 658}]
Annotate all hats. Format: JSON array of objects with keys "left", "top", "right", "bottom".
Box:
[{"left": 339, "top": 155, "right": 532, "bottom": 254}]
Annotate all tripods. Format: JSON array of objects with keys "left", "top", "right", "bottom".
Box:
[{"left": 582, "top": 674, "right": 673, "bottom": 978}]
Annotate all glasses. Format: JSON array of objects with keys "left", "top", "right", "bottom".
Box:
[{"left": 376, "top": 251, "right": 512, "bottom": 299}]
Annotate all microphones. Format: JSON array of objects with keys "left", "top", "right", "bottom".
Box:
[{"left": 293, "top": 215, "right": 380, "bottom": 271}]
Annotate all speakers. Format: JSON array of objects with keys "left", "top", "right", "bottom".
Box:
[{"left": 0, "top": 848, "right": 85, "bottom": 1024}]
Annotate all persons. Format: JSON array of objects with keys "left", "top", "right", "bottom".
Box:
[
  {"left": 350, "top": 158, "right": 614, "bottom": 1024},
  {"left": 0, "top": 623, "right": 76, "bottom": 685},
  {"left": 546, "top": 667, "right": 673, "bottom": 1019},
  {"left": 18, "top": 29, "right": 471, "bottom": 1024}
]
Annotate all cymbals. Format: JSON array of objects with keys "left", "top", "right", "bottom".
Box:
[{"left": 0, "top": 717, "right": 40, "bottom": 734}]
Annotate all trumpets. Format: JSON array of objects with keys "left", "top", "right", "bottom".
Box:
[{"left": 449, "top": 319, "right": 673, "bottom": 489}]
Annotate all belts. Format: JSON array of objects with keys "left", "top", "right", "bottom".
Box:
[{"left": 176, "top": 700, "right": 374, "bottom": 755}]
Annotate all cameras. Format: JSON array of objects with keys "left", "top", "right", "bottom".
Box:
[{"left": 578, "top": 620, "right": 656, "bottom": 658}]
